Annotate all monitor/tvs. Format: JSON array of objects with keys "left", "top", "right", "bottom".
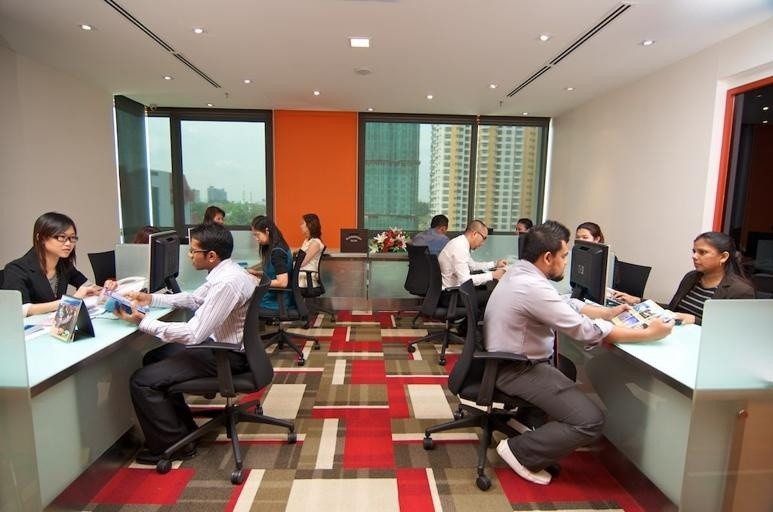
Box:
[
  {"left": 341, "top": 229, "right": 368, "bottom": 252},
  {"left": 570, "top": 240, "right": 608, "bottom": 305},
  {"left": 745, "top": 232, "right": 773, "bottom": 257},
  {"left": 149, "top": 229, "right": 181, "bottom": 294},
  {"left": 518, "top": 232, "right": 531, "bottom": 260},
  {"left": 755, "top": 240, "right": 773, "bottom": 272}
]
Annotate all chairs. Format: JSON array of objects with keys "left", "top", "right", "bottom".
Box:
[
  {"left": 84, "top": 249, "right": 117, "bottom": 289},
  {"left": 611, "top": 254, "right": 653, "bottom": 304},
  {"left": 735, "top": 225, "right": 772, "bottom": 301}
]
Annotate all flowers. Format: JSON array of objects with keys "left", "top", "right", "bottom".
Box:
[{"left": 365, "top": 226, "right": 412, "bottom": 257}]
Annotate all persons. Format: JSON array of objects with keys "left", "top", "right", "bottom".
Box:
[
  {"left": 96, "top": 287, "right": 146, "bottom": 317},
  {"left": 612, "top": 231, "right": 756, "bottom": 327},
  {"left": 437, "top": 219, "right": 508, "bottom": 338},
  {"left": 484, "top": 221, "right": 672, "bottom": 487},
  {"left": 292, "top": 213, "right": 325, "bottom": 288},
  {"left": 514, "top": 218, "right": 533, "bottom": 233},
  {"left": 113, "top": 222, "right": 260, "bottom": 464},
  {"left": 202, "top": 205, "right": 225, "bottom": 224},
  {"left": 247, "top": 215, "right": 265, "bottom": 277},
  {"left": 414, "top": 214, "right": 450, "bottom": 255},
  {"left": 0, "top": 212, "right": 118, "bottom": 318},
  {"left": 253, "top": 216, "right": 294, "bottom": 311},
  {"left": 576, "top": 222, "right": 621, "bottom": 289},
  {"left": 133, "top": 225, "right": 161, "bottom": 244}
]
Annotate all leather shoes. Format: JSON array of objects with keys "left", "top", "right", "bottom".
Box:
[{"left": 135, "top": 422, "right": 203, "bottom": 466}]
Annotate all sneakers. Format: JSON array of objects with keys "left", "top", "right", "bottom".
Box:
[{"left": 496, "top": 414, "right": 553, "bottom": 487}]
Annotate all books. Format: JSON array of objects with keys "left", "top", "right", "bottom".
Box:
[
  {"left": 50, "top": 294, "right": 83, "bottom": 342},
  {"left": 610, "top": 298, "right": 677, "bottom": 330}
]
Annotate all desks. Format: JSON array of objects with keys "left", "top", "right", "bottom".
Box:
[
  {"left": 0, "top": 277, "right": 186, "bottom": 510},
  {"left": 0, "top": 268, "right": 9, "bottom": 290},
  {"left": 320, "top": 247, "right": 413, "bottom": 316},
  {"left": 550, "top": 283, "right": 773, "bottom": 511}
]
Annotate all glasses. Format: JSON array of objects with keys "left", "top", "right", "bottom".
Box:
[
  {"left": 44, "top": 231, "right": 79, "bottom": 244},
  {"left": 188, "top": 246, "right": 212, "bottom": 255},
  {"left": 477, "top": 232, "right": 488, "bottom": 241}
]
